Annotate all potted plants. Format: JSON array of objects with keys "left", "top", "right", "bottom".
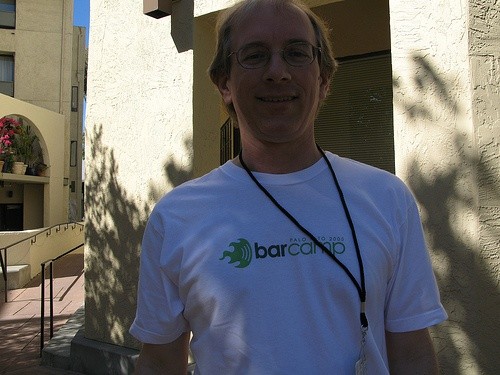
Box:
[{"left": 0, "top": 116, "right": 48, "bottom": 176}]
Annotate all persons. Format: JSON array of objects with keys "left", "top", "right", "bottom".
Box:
[{"left": 130, "top": 0, "right": 448, "bottom": 375}]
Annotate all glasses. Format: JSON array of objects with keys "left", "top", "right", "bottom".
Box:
[{"left": 227, "top": 42, "right": 322, "bottom": 70}]
